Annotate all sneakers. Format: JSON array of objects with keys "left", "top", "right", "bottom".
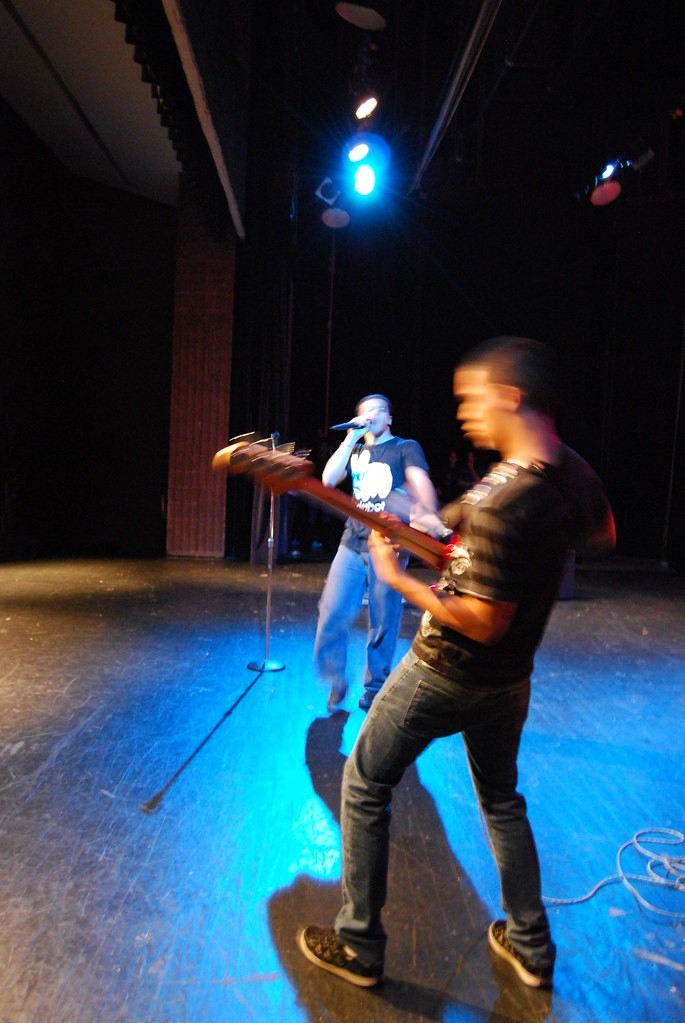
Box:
[
  {"left": 300, "top": 924, "right": 386, "bottom": 987},
  {"left": 488, "top": 920, "right": 553, "bottom": 987}
]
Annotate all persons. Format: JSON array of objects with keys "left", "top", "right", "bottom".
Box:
[
  {"left": 301, "top": 333, "right": 622, "bottom": 991},
  {"left": 317, "top": 395, "right": 443, "bottom": 711}
]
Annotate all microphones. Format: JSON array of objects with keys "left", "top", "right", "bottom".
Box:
[{"left": 331, "top": 422, "right": 365, "bottom": 431}]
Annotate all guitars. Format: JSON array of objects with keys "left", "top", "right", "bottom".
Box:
[{"left": 210, "top": 433, "right": 461, "bottom": 596}]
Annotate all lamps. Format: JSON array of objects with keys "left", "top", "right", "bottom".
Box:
[
  {"left": 586, "top": 177, "right": 621, "bottom": 207},
  {"left": 321, "top": 202, "right": 352, "bottom": 228},
  {"left": 344, "top": 74, "right": 379, "bottom": 120}
]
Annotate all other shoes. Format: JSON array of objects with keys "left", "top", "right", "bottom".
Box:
[
  {"left": 359, "top": 692, "right": 378, "bottom": 707},
  {"left": 328, "top": 682, "right": 349, "bottom": 704}
]
